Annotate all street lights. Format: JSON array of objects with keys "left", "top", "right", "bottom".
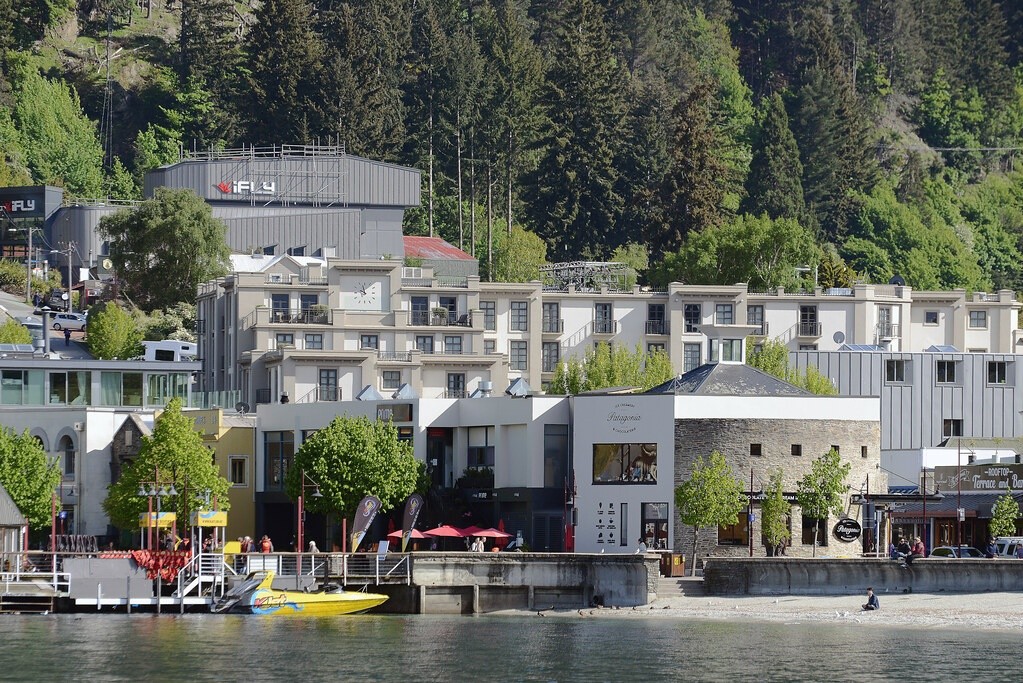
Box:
[
  {"left": 60, "top": 474, "right": 79, "bottom": 535},
  {"left": 565, "top": 477, "right": 576, "bottom": 552},
  {"left": 857, "top": 473, "right": 872, "bottom": 558},
  {"left": 184, "top": 472, "right": 206, "bottom": 551},
  {"left": 301, "top": 468, "right": 324, "bottom": 553},
  {"left": 957, "top": 438, "right": 978, "bottom": 559},
  {"left": 137, "top": 462, "right": 179, "bottom": 551},
  {"left": 8, "top": 227, "right": 32, "bottom": 303},
  {"left": 50, "top": 240, "right": 72, "bottom": 313},
  {"left": 923, "top": 467, "right": 946, "bottom": 557},
  {"left": 750, "top": 467, "right": 769, "bottom": 557}
]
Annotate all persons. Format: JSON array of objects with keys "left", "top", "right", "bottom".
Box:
[
  {"left": 106, "top": 542, "right": 114, "bottom": 551},
  {"left": 986, "top": 536, "right": 1000, "bottom": 558},
  {"left": 862, "top": 587, "right": 879, "bottom": 612},
  {"left": 158, "top": 529, "right": 274, "bottom": 575},
  {"left": 468, "top": 535, "right": 486, "bottom": 552},
  {"left": 890, "top": 535, "right": 925, "bottom": 569},
  {"left": 308, "top": 540, "right": 321, "bottom": 553},
  {"left": 638, "top": 538, "right": 646, "bottom": 552},
  {"left": 1014, "top": 544, "right": 1023, "bottom": 559},
  {"left": 64, "top": 328, "right": 71, "bottom": 346}
]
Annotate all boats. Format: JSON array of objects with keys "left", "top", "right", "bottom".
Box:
[{"left": 209, "top": 567, "right": 391, "bottom": 618}]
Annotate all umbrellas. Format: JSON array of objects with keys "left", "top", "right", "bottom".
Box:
[
  {"left": 387, "top": 526, "right": 431, "bottom": 539},
  {"left": 422, "top": 523, "right": 514, "bottom": 538}
]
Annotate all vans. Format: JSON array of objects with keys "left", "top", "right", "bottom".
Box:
[{"left": 992, "top": 536, "right": 1023, "bottom": 559}]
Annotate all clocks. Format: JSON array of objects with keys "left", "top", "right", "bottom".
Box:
[{"left": 337, "top": 272, "right": 393, "bottom": 314}]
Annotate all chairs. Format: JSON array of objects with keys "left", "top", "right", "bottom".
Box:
[{"left": 453, "top": 314, "right": 468, "bottom": 326}]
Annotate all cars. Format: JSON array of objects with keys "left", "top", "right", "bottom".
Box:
[
  {"left": 928, "top": 543, "right": 987, "bottom": 558},
  {"left": 52, "top": 310, "right": 89, "bottom": 333}
]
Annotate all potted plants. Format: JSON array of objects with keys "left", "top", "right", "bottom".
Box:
[
  {"left": 311, "top": 305, "right": 328, "bottom": 324},
  {"left": 432, "top": 307, "right": 448, "bottom": 326}
]
